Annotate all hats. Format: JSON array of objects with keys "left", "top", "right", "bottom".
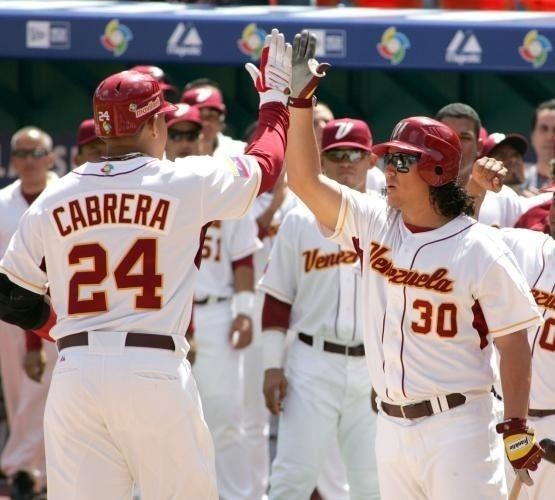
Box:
[
  {"left": 77, "top": 66, "right": 225, "bottom": 144},
  {"left": 484, "top": 133, "right": 527, "bottom": 156},
  {"left": 320, "top": 119, "right": 372, "bottom": 153}
]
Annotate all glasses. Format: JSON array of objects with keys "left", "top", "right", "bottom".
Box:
[
  {"left": 12, "top": 149, "right": 48, "bottom": 157},
  {"left": 383, "top": 153, "right": 418, "bottom": 168},
  {"left": 168, "top": 130, "right": 199, "bottom": 140},
  {"left": 326, "top": 149, "right": 365, "bottom": 161}
]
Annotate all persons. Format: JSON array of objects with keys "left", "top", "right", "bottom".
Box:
[{"left": 0, "top": 28, "right": 555, "bottom": 500}]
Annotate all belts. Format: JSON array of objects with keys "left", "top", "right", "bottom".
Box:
[
  {"left": 57, "top": 331, "right": 175, "bottom": 352},
  {"left": 299, "top": 333, "right": 364, "bottom": 355},
  {"left": 491, "top": 385, "right": 555, "bottom": 417},
  {"left": 371, "top": 388, "right": 465, "bottom": 419}
]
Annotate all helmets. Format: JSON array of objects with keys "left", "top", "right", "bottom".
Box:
[{"left": 372, "top": 116, "right": 462, "bottom": 187}]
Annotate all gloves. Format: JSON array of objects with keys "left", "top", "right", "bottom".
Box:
[
  {"left": 496, "top": 418, "right": 545, "bottom": 485},
  {"left": 245, "top": 28, "right": 331, "bottom": 107}
]
